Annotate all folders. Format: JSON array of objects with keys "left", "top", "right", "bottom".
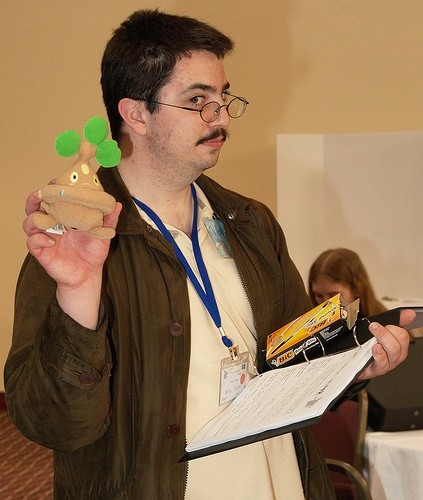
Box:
[{"left": 177, "top": 307, "right": 423, "bottom": 464}]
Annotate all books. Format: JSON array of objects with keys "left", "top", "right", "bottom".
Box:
[{"left": 177, "top": 333, "right": 380, "bottom": 463}]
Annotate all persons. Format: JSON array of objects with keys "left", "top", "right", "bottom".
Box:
[
  {"left": 308, "top": 248, "right": 387, "bottom": 317},
  {"left": 4, "top": 6, "right": 416, "bottom": 500}
]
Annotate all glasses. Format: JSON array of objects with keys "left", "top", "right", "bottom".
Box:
[{"left": 134, "top": 94, "right": 249, "bottom": 124}]
